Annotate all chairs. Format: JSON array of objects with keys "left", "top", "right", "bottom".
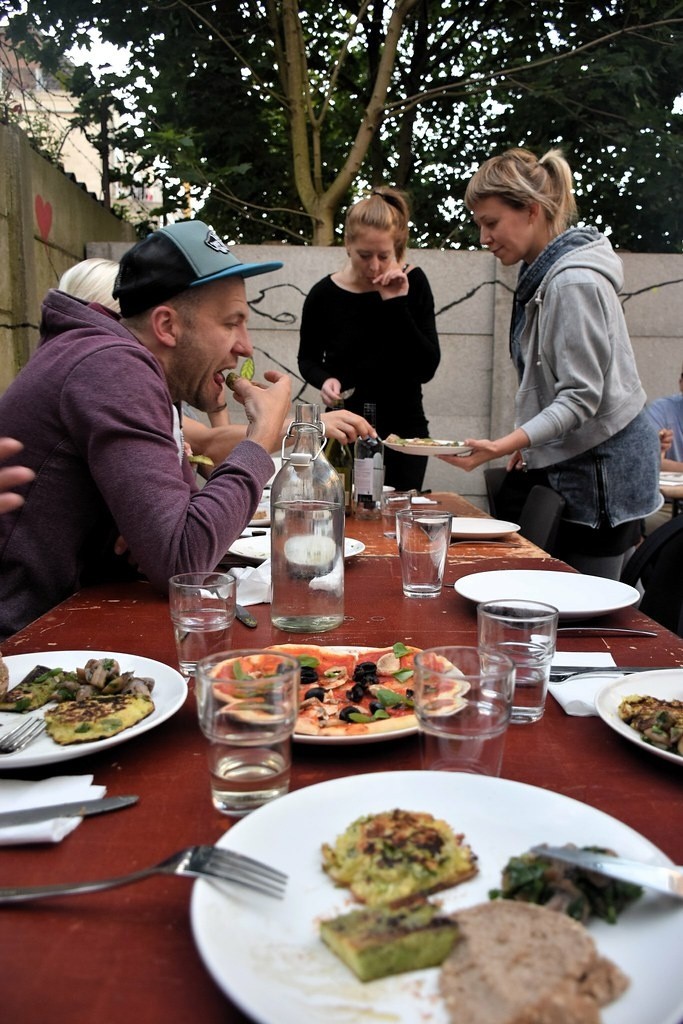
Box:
[
  {"left": 623, "top": 509, "right": 683, "bottom": 636},
  {"left": 483, "top": 467, "right": 560, "bottom": 550}
]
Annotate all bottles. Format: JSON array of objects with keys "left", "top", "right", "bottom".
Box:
[
  {"left": 271, "top": 403, "right": 346, "bottom": 634},
  {"left": 325, "top": 398, "right": 385, "bottom": 519}
]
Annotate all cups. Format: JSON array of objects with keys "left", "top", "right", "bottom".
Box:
[
  {"left": 196, "top": 648, "right": 301, "bottom": 816},
  {"left": 380, "top": 492, "right": 453, "bottom": 599},
  {"left": 170, "top": 570, "right": 237, "bottom": 676},
  {"left": 477, "top": 599, "right": 559, "bottom": 724},
  {"left": 413, "top": 645, "right": 517, "bottom": 775}
]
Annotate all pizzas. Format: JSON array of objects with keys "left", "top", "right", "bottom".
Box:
[{"left": 210, "top": 643, "right": 462, "bottom": 735}]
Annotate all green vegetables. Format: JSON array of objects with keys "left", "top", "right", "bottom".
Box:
[{"left": 488, "top": 846, "right": 643, "bottom": 924}]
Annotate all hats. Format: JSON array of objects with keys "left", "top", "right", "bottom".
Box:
[{"left": 112, "top": 219, "right": 284, "bottom": 320}]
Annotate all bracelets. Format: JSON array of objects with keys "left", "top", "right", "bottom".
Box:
[{"left": 209, "top": 402, "right": 227, "bottom": 415}]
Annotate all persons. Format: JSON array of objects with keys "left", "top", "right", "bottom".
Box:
[
  {"left": 434, "top": 146, "right": 664, "bottom": 581},
  {"left": 297, "top": 186, "right": 441, "bottom": 492},
  {"left": 0, "top": 220, "right": 291, "bottom": 641},
  {"left": 57, "top": 257, "right": 378, "bottom": 479},
  {"left": 1, "top": 437, "right": 35, "bottom": 515},
  {"left": 642, "top": 365, "right": 683, "bottom": 539}
]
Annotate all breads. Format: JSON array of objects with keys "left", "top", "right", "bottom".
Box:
[{"left": 442, "top": 901, "right": 631, "bottom": 1024}]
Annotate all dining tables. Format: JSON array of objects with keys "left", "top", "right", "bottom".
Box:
[{"left": 0, "top": 493, "right": 683, "bottom": 1024}]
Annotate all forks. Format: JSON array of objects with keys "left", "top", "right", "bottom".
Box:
[
  {"left": 0, "top": 718, "right": 48, "bottom": 754},
  {"left": 0, "top": 846, "right": 288, "bottom": 904}
]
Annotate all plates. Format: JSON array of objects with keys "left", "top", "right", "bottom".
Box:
[
  {"left": 595, "top": 669, "right": 683, "bottom": 765},
  {"left": 454, "top": 568, "right": 641, "bottom": 622},
  {"left": 383, "top": 438, "right": 474, "bottom": 456},
  {"left": 227, "top": 457, "right": 366, "bottom": 558},
  {"left": 353, "top": 485, "right": 521, "bottom": 540},
  {"left": 0, "top": 651, "right": 188, "bottom": 768},
  {"left": 209, "top": 644, "right": 470, "bottom": 742},
  {"left": 190, "top": 771, "right": 683, "bottom": 1024}
]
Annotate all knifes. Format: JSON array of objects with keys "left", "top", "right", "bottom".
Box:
[
  {"left": 533, "top": 846, "right": 683, "bottom": 902},
  {"left": 236, "top": 604, "right": 257, "bottom": 629},
  {"left": 0, "top": 795, "right": 139, "bottom": 830}
]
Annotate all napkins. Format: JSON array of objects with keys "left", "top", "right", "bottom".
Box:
[
  {"left": 411, "top": 497, "right": 438, "bottom": 504},
  {"left": 199, "top": 559, "right": 271, "bottom": 606},
  {"left": 0, "top": 775, "right": 109, "bottom": 843},
  {"left": 547, "top": 652, "right": 627, "bottom": 716}
]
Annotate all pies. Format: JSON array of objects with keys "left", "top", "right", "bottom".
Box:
[
  {"left": 618, "top": 694, "right": 683, "bottom": 756},
  {"left": 0, "top": 658, "right": 156, "bottom": 745},
  {"left": 387, "top": 433, "right": 444, "bottom": 446},
  {"left": 318, "top": 810, "right": 479, "bottom": 981}
]
